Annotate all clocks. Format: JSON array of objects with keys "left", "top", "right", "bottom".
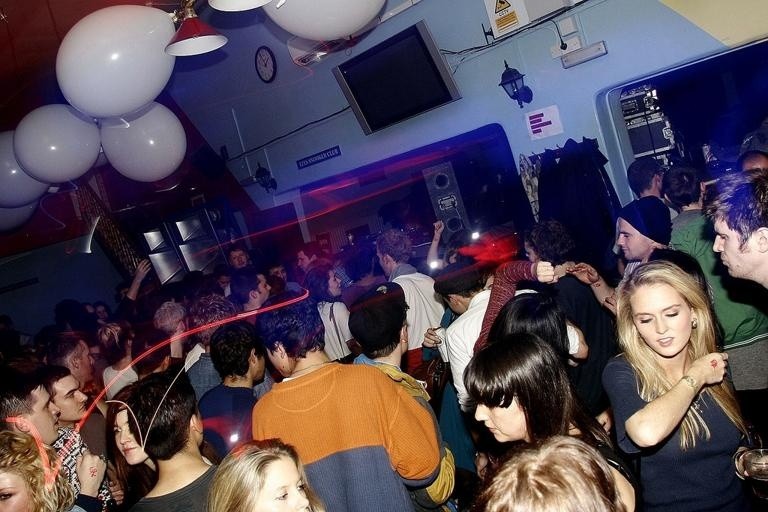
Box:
[{"left": 255, "top": 46, "right": 277, "bottom": 83}]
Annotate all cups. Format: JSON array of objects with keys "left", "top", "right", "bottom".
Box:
[{"left": 743, "top": 449, "right": 768, "bottom": 500}]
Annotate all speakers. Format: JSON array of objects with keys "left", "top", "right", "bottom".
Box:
[{"left": 422, "top": 160, "right": 472, "bottom": 245}]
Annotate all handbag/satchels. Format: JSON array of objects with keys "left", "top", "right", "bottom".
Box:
[{"left": 410, "top": 358, "right": 446, "bottom": 405}]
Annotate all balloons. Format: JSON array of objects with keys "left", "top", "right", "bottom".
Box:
[
  {"left": 100, "top": 103, "right": 188, "bottom": 183},
  {"left": 0, "top": 103, "right": 102, "bottom": 232},
  {"left": 55, "top": 5, "right": 182, "bottom": 119},
  {"left": 265, "top": 0, "right": 384, "bottom": 41}
]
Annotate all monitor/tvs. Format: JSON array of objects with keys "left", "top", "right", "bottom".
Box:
[{"left": 331, "top": 18, "right": 463, "bottom": 136}]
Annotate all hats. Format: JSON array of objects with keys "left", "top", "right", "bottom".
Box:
[{"left": 618, "top": 196, "right": 673, "bottom": 246}]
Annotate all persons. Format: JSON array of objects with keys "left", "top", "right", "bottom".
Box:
[{"left": 2, "top": 149, "right": 768, "bottom": 511}]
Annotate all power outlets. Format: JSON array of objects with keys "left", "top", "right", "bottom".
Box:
[{"left": 549, "top": 36, "right": 582, "bottom": 59}]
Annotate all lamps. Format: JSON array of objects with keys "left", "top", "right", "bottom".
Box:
[
  {"left": 164, "top": 0, "right": 229, "bottom": 57},
  {"left": 255, "top": 161, "right": 278, "bottom": 195},
  {"left": 497, "top": 59, "right": 533, "bottom": 109}
]
